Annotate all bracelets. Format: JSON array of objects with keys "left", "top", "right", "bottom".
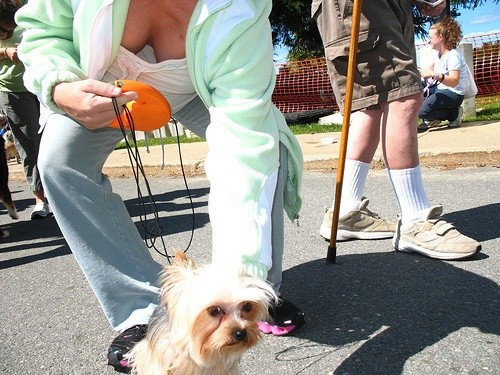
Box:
[{"left": 5, "top": 46, "right": 8, "bottom": 57}]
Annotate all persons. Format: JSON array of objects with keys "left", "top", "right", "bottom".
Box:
[
  {"left": 309, "top": 0, "right": 482, "bottom": 261},
  {"left": 0, "top": 0, "right": 48, "bottom": 218},
  {"left": 415, "top": 16, "right": 478, "bottom": 131},
  {"left": 13, "top": 0, "right": 303, "bottom": 370}
]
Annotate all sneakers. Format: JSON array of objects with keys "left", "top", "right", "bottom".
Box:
[
  {"left": 319, "top": 196, "right": 396, "bottom": 241},
  {"left": 258, "top": 296, "right": 305, "bottom": 336},
  {"left": 391, "top": 204, "right": 481, "bottom": 260},
  {"left": 106, "top": 324, "right": 147, "bottom": 369}
]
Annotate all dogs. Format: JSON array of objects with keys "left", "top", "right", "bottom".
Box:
[{"left": 122, "top": 251, "right": 280, "bottom": 375}]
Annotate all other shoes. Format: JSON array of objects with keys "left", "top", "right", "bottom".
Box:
[
  {"left": 448, "top": 106, "right": 463, "bottom": 128},
  {"left": 417, "top": 119, "right": 441, "bottom": 130},
  {"left": 31, "top": 202, "right": 49, "bottom": 219}
]
